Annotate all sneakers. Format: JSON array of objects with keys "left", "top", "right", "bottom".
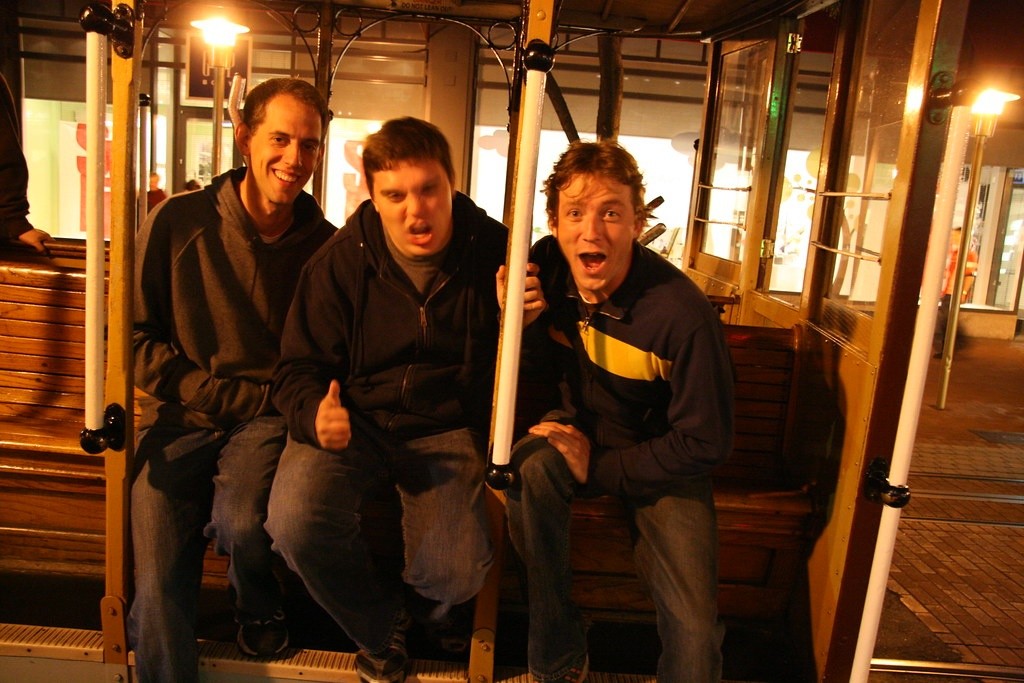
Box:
[
  {"left": 353, "top": 610, "right": 412, "bottom": 683},
  {"left": 233, "top": 605, "right": 289, "bottom": 655}
]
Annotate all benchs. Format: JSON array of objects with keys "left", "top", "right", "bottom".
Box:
[
  {"left": 0, "top": 263, "right": 812, "bottom": 623},
  {"left": 14, "top": 239, "right": 110, "bottom": 271}
]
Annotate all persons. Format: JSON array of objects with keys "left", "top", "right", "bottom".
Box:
[
  {"left": 270, "top": 117, "right": 547, "bottom": 682},
  {"left": 129, "top": 76, "right": 338, "bottom": 682},
  {"left": 0, "top": 70, "right": 57, "bottom": 258},
  {"left": 499, "top": 139, "right": 738, "bottom": 683}
]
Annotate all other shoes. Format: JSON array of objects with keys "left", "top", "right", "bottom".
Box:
[{"left": 533, "top": 652, "right": 589, "bottom": 683}]
